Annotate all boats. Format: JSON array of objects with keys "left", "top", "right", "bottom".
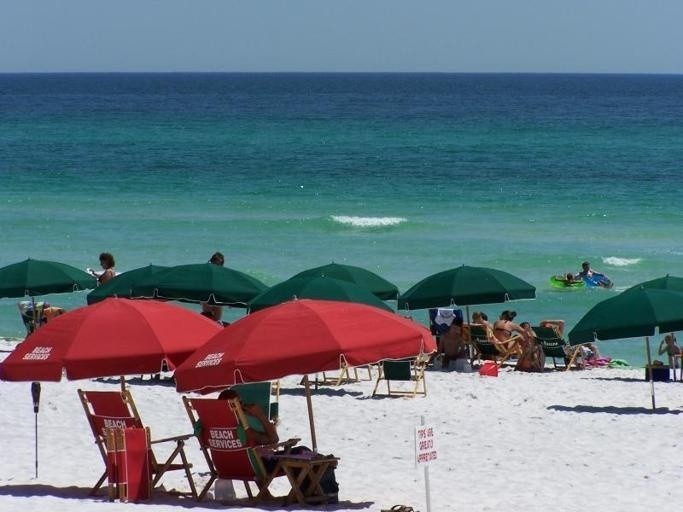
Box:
[{"left": 549, "top": 273, "right": 613, "bottom": 288}]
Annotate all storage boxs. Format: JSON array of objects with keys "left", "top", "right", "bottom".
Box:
[{"left": 646, "top": 365, "right": 669, "bottom": 381}]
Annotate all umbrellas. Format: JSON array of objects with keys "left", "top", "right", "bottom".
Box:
[
  {"left": 620, "top": 273, "right": 682, "bottom": 293},
  {"left": 567, "top": 284, "right": 683, "bottom": 407}
]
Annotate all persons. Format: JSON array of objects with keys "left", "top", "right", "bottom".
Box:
[
  {"left": 539, "top": 319, "right": 599, "bottom": 358},
  {"left": 88, "top": 253, "right": 114, "bottom": 283},
  {"left": 582, "top": 261, "right": 612, "bottom": 288},
  {"left": 437, "top": 310, "right": 536, "bottom": 369},
  {"left": 658, "top": 335, "right": 680, "bottom": 356},
  {"left": 200, "top": 252, "right": 223, "bottom": 320},
  {"left": 555, "top": 272, "right": 575, "bottom": 286},
  {"left": 191, "top": 388, "right": 311, "bottom": 471}
]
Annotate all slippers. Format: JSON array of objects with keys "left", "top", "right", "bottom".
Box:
[{"left": 380, "top": 504, "right": 413, "bottom": 512}]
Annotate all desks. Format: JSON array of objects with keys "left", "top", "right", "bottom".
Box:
[{"left": 275, "top": 455, "right": 341, "bottom": 506}]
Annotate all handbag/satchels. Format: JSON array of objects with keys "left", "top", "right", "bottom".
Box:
[{"left": 513, "top": 341, "right": 546, "bottom": 373}]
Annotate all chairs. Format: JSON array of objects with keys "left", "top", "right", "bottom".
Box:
[
  {"left": 77, "top": 389, "right": 198, "bottom": 503},
  {"left": 182, "top": 396, "right": 301, "bottom": 504},
  {"left": 18, "top": 302, "right": 66, "bottom": 337},
  {"left": 672, "top": 347, "right": 683, "bottom": 382},
  {"left": 300, "top": 315, "right": 585, "bottom": 398}
]
